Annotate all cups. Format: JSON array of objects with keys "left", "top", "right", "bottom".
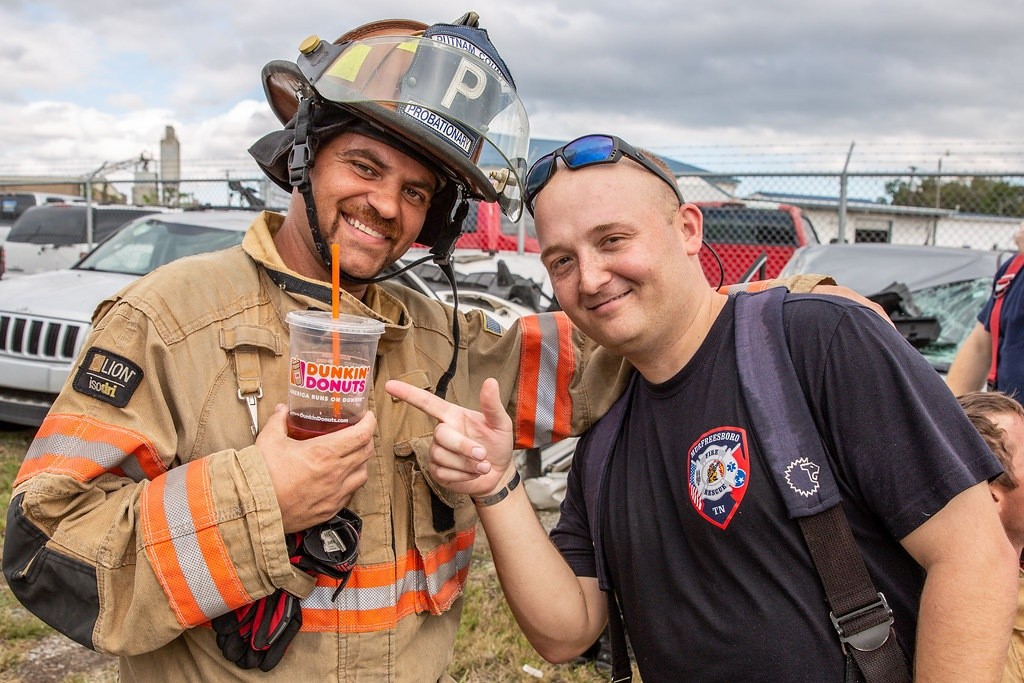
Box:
[{"left": 284, "top": 309, "right": 386, "bottom": 442}]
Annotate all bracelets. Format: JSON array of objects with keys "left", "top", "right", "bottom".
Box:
[{"left": 470, "top": 471, "right": 521, "bottom": 506}]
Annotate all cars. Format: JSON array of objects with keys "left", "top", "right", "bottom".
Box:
[{"left": 0, "top": 193, "right": 1016, "bottom": 506}]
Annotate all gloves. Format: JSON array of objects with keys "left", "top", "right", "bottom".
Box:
[{"left": 212, "top": 508, "right": 362, "bottom": 671}]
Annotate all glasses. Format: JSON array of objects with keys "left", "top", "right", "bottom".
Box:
[{"left": 523, "top": 134, "right": 685, "bottom": 219}]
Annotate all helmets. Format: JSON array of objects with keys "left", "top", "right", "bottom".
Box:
[{"left": 247, "top": 11, "right": 530, "bottom": 224}]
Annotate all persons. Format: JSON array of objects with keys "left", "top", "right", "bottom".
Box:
[
  {"left": 2, "top": 13, "right": 897, "bottom": 683},
  {"left": 385, "top": 145, "right": 1024, "bottom": 683},
  {"left": 958, "top": 392, "right": 1024, "bottom": 683},
  {"left": 947, "top": 221, "right": 1024, "bottom": 398}
]
